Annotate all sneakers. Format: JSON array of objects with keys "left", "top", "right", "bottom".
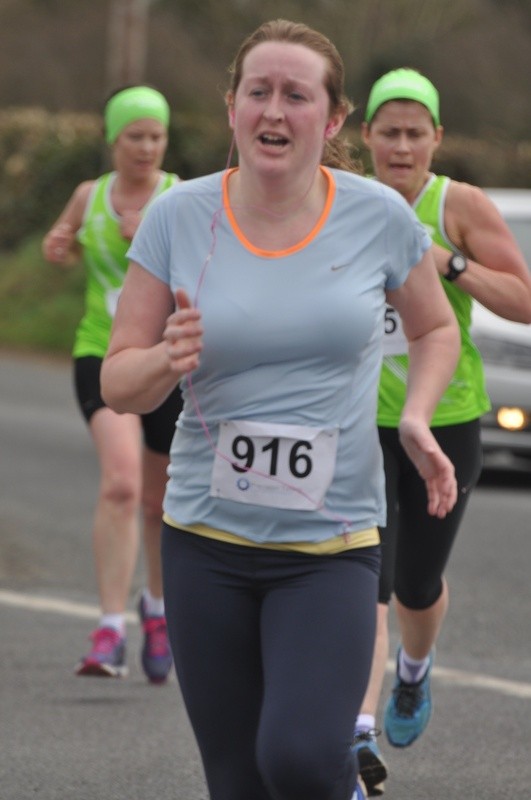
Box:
[
  {"left": 382, "top": 642, "right": 436, "bottom": 748},
  {"left": 73, "top": 624, "right": 130, "bottom": 678},
  {"left": 350, "top": 726, "right": 388, "bottom": 798},
  {"left": 136, "top": 589, "right": 174, "bottom": 684}
]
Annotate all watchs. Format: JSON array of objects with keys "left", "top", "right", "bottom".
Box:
[{"left": 444, "top": 254, "right": 468, "bottom": 282}]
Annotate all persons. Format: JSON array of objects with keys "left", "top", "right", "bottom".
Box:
[
  {"left": 41, "top": 84, "right": 186, "bottom": 686},
  {"left": 99, "top": 20, "right": 462, "bottom": 800},
  {"left": 352, "top": 67, "right": 531, "bottom": 797}
]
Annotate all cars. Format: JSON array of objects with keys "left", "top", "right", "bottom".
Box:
[{"left": 467, "top": 187, "right": 531, "bottom": 470}]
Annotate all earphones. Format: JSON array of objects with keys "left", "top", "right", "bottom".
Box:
[
  {"left": 325, "top": 120, "right": 335, "bottom": 136},
  {"left": 231, "top": 111, "right": 235, "bottom": 128}
]
